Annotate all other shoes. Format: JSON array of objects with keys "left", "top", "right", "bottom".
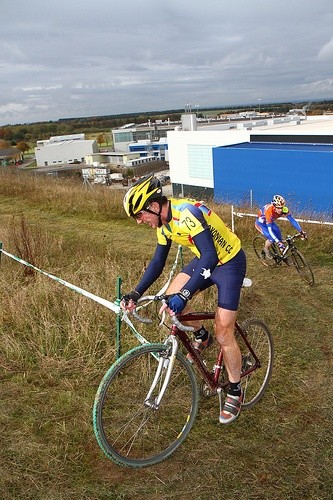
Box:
[
  {"left": 283, "top": 258, "right": 291, "bottom": 266},
  {"left": 264, "top": 248, "right": 273, "bottom": 260}
]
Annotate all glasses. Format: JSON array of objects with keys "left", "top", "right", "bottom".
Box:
[{"left": 134, "top": 204, "right": 153, "bottom": 219}]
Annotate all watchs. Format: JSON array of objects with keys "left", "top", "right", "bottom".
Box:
[{"left": 180, "top": 287, "right": 192, "bottom": 299}]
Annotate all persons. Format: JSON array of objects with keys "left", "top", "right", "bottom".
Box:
[
  {"left": 120, "top": 173, "right": 248, "bottom": 423},
  {"left": 255, "top": 195, "right": 308, "bottom": 261}
]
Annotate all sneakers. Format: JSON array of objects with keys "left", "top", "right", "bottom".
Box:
[
  {"left": 219, "top": 388, "right": 243, "bottom": 423},
  {"left": 186, "top": 332, "right": 214, "bottom": 364}
]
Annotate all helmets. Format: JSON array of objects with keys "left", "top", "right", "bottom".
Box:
[
  {"left": 272, "top": 194, "right": 285, "bottom": 208},
  {"left": 123, "top": 174, "right": 162, "bottom": 217}
]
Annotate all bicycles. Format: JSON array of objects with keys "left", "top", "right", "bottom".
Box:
[
  {"left": 253, "top": 230, "right": 315, "bottom": 286},
  {"left": 93, "top": 292, "right": 274, "bottom": 469}
]
